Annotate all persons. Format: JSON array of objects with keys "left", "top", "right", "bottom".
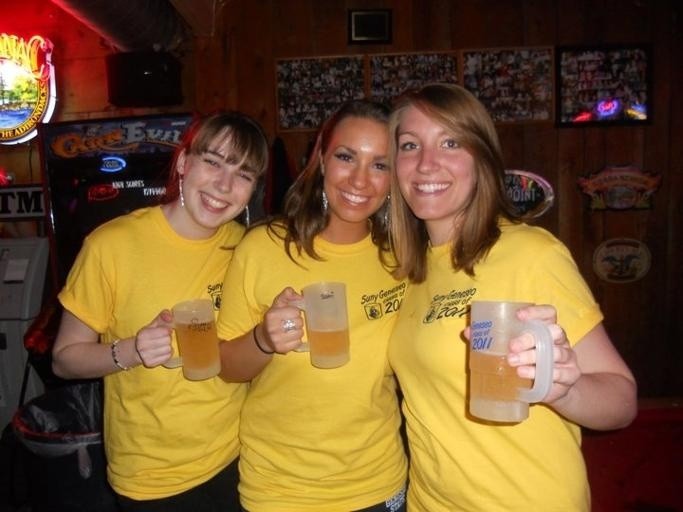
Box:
[
  {"left": 387, "top": 82, "right": 642, "bottom": 511},
  {"left": 50, "top": 107, "right": 275, "bottom": 511},
  {"left": 217, "top": 95, "right": 409, "bottom": 512}
]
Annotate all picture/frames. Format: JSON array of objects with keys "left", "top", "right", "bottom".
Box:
[
  {"left": 270, "top": 53, "right": 368, "bottom": 135},
  {"left": 555, "top": 38, "right": 656, "bottom": 128},
  {"left": 367, "top": 48, "right": 461, "bottom": 109},
  {"left": 461, "top": 40, "right": 557, "bottom": 125}
]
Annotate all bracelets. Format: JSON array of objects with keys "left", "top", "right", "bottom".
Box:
[
  {"left": 110, "top": 339, "right": 129, "bottom": 372},
  {"left": 251, "top": 323, "right": 276, "bottom": 359}
]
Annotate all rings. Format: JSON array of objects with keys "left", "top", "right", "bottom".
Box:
[{"left": 281, "top": 316, "right": 296, "bottom": 334}]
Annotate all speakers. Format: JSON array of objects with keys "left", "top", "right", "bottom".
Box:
[{"left": 106, "top": 49, "right": 185, "bottom": 108}]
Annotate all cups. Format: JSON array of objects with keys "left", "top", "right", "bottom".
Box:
[
  {"left": 466, "top": 298, "right": 555, "bottom": 429},
  {"left": 286, "top": 281, "right": 354, "bottom": 373},
  {"left": 152, "top": 298, "right": 227, "bottom": 383}
]
